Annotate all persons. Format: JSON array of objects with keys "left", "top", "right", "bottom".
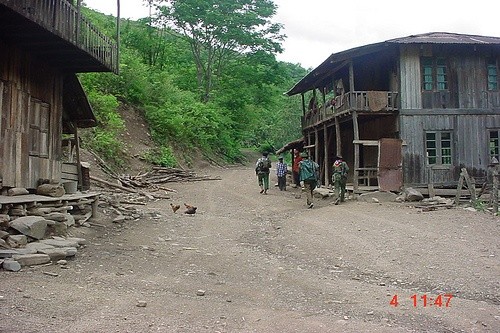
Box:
[
  {"left": 298, "top": 152, "right": 319, "bottom": 208},
  {"left": 256, "top": 152, "right": 271, "bottom": 195},
  {"left": 332, "top": 153, "right": 349, "bottom": 205},
  {"left": 277, "top": 157, "right": 287, "bottom": 191},
  {"left": 293, "top": 150, "right": 303, "bottom": 188},
  {"left": 309, "top": 90, "right": 319, "bottom": 114}
]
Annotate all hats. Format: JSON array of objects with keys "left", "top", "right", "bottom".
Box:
[
  {"left": 336, "top": 153, "right": 343, "bottom": 159},
  {"left": 300, "top": 152, "right": 307, "bottom": 158},
  {"left": 278, "top": 157, "right": 283, "bottom": 160}
]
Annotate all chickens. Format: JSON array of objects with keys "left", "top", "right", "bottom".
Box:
[
  {"left": 184, "top": 203, "right": 198, "bottom": 214},
  {"left": 170, "top": 203, "right": 180, "bottom": 213}
]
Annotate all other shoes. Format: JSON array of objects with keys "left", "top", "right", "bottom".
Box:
[
  {"left": 335, "top": 199, "right": 340, "bottom": 205},
  {"left": 309, "top": 203, "right": 314, "bottom": 209},
  {"left": 260, "top": 189, "right": 264, "bottom": 193}
]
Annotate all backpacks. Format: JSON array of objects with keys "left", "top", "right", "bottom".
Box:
[{"left": 258, "top": 158, "right": 270, "bottom": 172}]
